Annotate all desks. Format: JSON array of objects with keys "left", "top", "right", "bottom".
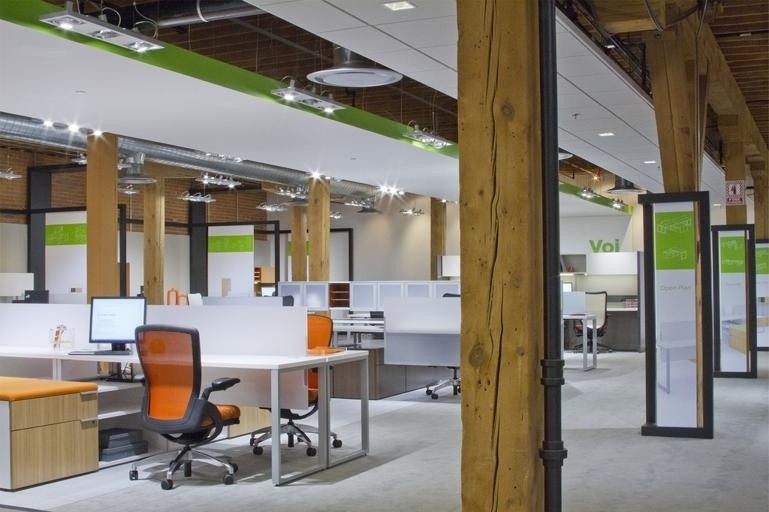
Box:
[
  {"left": 0, "top": 345, "right": 59, "bottom": 382},
  {"left": 600, "top": 295, "right": 644, "bottom": 360},
  {"left": 55, "top": 344, "right": 328, "bottom": 486},
  {"left": 328, "top": 318, "right": 470, "bottom": 349},
  {"left": 321, "top": 349, "right": 374, "bottom": 470},
  {"left": 558, "top": 310, "right": 597, "bottom": 376}
]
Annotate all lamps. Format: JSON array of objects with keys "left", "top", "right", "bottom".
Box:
[
  {"left": 268, "top": 21, "right": 349, "bottom": 115},
  {"left": 397, "top": 75, "right": 454, "bottom": 150},
  {"left": 2, "top": 119, "right": 314, "bottom": 214},
  {"left": 39, "top": 1, "right": 165, "bottom": 55},
  {"left": 314, "top": 171, "right": 460, "bottom": 218},
  {"left": 559, "top": 129, "right": 648, "bottom": 212}
]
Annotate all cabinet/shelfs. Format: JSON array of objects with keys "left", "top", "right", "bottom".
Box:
[
  {"left": 251, "top": 262, "right": 275, "bottom": 294},
  {"left": 0, "top": 371, "right": 101, "bottom": 494}
]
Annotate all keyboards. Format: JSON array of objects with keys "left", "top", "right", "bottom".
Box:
[{"left": 69, "top": 350, "right": 130, "bottom": 355}]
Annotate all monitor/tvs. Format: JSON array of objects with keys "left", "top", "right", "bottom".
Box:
[{"left": 89, "top": 296, "right": 146, "bottom": 350}]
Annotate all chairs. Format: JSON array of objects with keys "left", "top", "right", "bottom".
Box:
[
  {"left": 570, "top": 289, "right": 614, "bottom": 356},
  {"left": 250, "top": 310, "right": 342, "bottom": 459},
  {"left": 423, "top": 290, "right": 465, "bottom": 406},
  {"left": 126, "top": 323, "right": 243, "bottom": 491}
]
